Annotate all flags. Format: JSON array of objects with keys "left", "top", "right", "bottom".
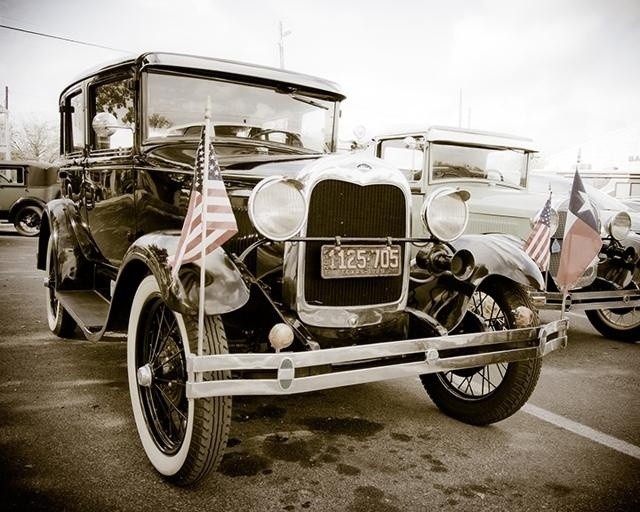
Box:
[
  {"left": 522, "top": 195, "right": 554, "bottom": 275},
  {"left": 166, "top": 126, "right": 238, "bottom": 283},
  {"left": 558, "top": 171, "right": 604, "bottom": 295}
]
